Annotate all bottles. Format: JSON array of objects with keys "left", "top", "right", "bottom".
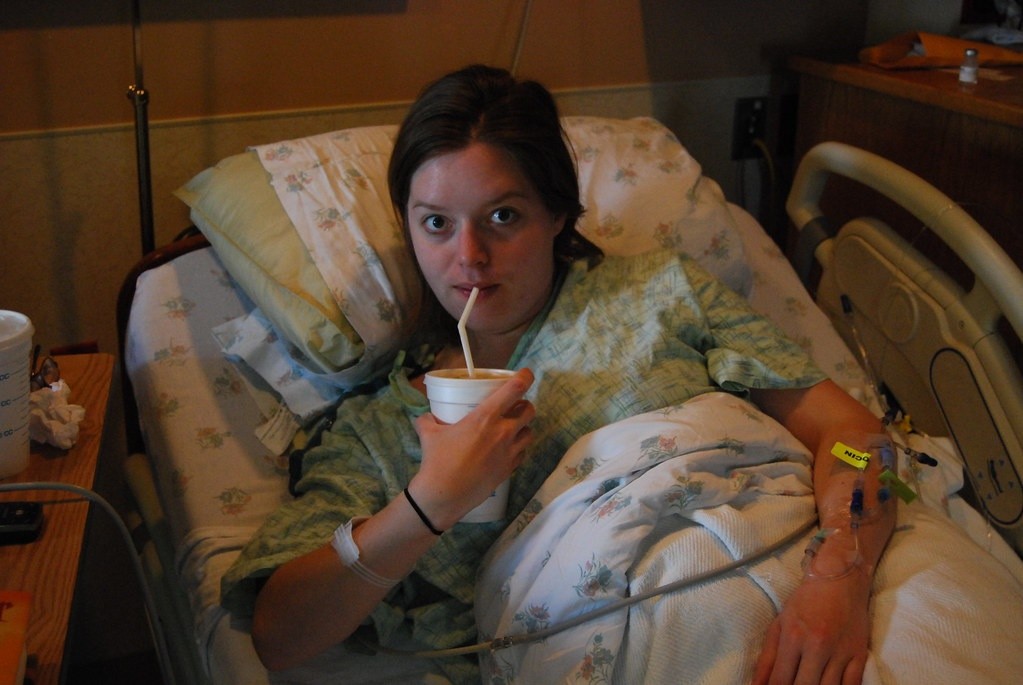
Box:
[{"left": 958, "top": 48, "right": 978, "bottom": 87}]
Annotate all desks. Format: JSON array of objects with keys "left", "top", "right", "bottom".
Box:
[{"left": 0, "top": 352, "right": 115, "bottom": 685}]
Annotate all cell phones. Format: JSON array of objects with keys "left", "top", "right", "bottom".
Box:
[{"left": 0, "top": 501, "right": 43, "bottom": 545}]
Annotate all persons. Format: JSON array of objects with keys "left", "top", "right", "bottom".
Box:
[{"left": 219, "top": 63, "right": 1019, "bottom": 685}]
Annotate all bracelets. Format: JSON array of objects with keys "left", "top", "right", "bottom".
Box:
[{"left": 403, "top": 485, "right": 445, "bottom": 536}]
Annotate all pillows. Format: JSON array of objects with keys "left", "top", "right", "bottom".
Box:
[{"left": 173, "top": 114, "right": 751, "bottom": 390}]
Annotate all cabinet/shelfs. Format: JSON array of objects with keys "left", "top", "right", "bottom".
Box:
[{"left": 790, "top": 53, "right": 1023, "bottom": 292}]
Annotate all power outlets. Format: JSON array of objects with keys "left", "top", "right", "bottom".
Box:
[{"left": 730, "top": 97, "right": 803, "bottom": 163}]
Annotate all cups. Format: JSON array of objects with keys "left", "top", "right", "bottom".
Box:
[
  {"left": 0, "top": 308, "right": 32, "bottom": 482},
  {"left": 422, "top": 367, "right": 517, "bottom": 524}
]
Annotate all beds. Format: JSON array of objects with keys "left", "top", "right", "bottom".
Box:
[{"left": 115, "top": 139, "right": 1023, "bottom": 685}]
